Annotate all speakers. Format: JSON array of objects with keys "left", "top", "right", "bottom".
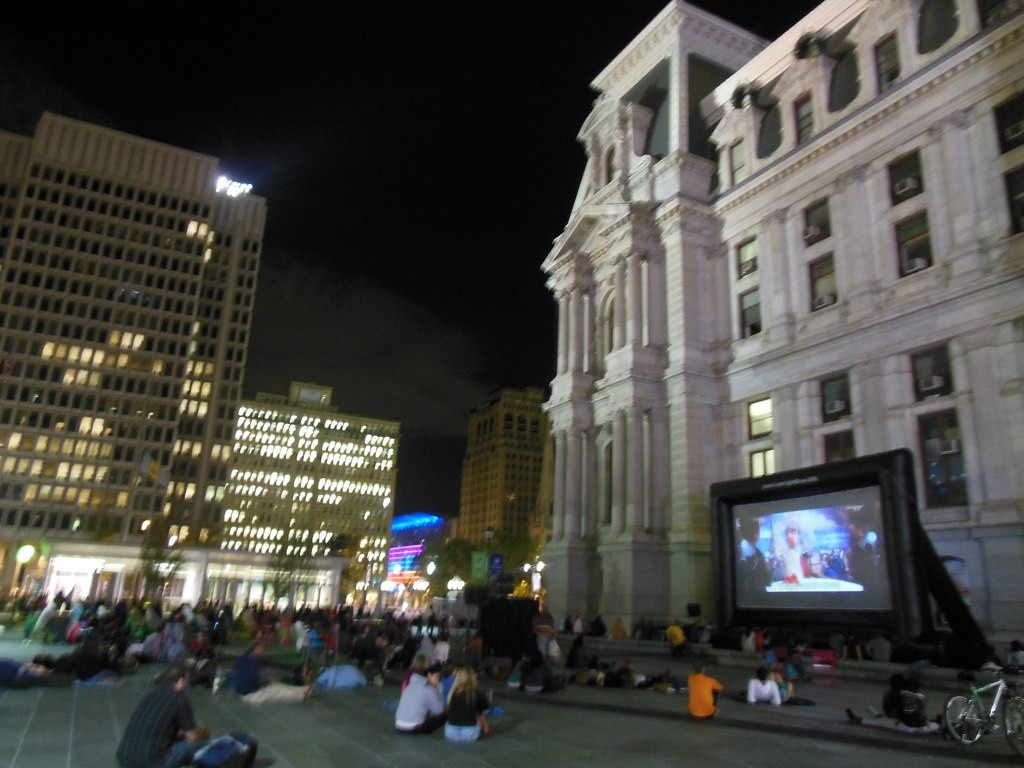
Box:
[
  {"left": 465, "top": 585, "right": 490, "bottom": 606},
  {"left": 686, "top": 603, "right": 701, "bottom": 617}
]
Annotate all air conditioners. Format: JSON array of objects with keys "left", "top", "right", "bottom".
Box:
[
  {"left": 814, "top": 296, "right": 835, "bottom": 308},
  {"left": 803, "top": 226, "right": 820, "bottom": 240},
  {"left": 826, "top": 400, "right": 846, "bottom": 413},
  {"left": 741, "top": 262, "right": 753, "bottom": 271},
  {"left": 895, "top": 178, "right": 919, "bottom": 196},
  {"left": 938, "top": 440, "right": 960, "bottom": 454},
  {"left": 904, "top": 257, "right": 928, "bottom": 273},
  {"left": 920, "top": 376, "right": 943, "bottom": 390},
  {"left": 1006, "top": 121, "right": 1024, "bottom": 139}
]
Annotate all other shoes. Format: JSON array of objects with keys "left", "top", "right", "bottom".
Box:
[
  {"left": 846, "top": 708, "right": 856, "bottom": 722},
  {"left": 491, "top": 706, "right": 506, "bottom": 716},
  {"left": 303, "top": 682, "right": 315, "bottom": 702}
]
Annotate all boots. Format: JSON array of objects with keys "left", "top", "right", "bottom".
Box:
[{"left": 934, "top": 713, "right": 943, "bottom": 724}]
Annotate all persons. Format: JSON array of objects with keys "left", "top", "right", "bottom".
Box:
[
  {"left": 115, "top": 664, "right": 212, "bottom": 768},
  {"left": 781, "top": 510, "right": 877, "bottom": 587},
  {"left": 0, "top": 585, "right": 688, "bottom": 742},
  {"left": 737, "top": 516, "right": 775, "bottom": 597},
  {"left": 728, "top": 619, "right": 900, "bottom": 668},
  {"left": 686, "top": 663, "right": 727, "bottom": 722},
  {"left": 746, "top": 664, "right": 794, "bottom": 706},
  {"left": 843, "top": 670, "right": 946, "bottom": 741}
]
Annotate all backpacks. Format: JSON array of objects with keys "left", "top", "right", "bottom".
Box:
[{"left": 192, "top": 730, "right": 257, "bottom": 768}]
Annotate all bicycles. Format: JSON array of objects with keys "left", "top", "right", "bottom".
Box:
[{"left": 945, "top": 665, "right": 1024, "bottom": 758}]
